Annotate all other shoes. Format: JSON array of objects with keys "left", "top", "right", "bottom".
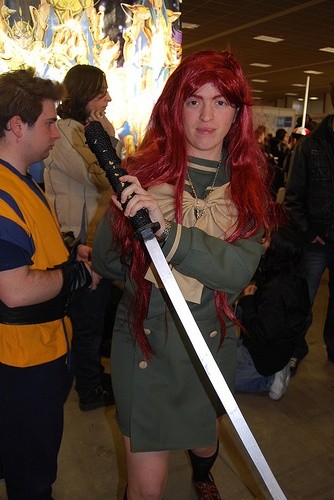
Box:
[
  {"left": 270, "top": 367, "right": 291, "bottom": 400},
  {"left": 191, "top": 470, "right": 222, "bottom": 500},
  {"left": 289, "top": 358, "right": 298, "bottom": 376},
  {"left": 77, "top": 366, "right": 116, "bottom": 411}
]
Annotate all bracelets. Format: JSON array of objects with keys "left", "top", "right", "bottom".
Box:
[{"left": 157, "top": 218, "right": 171, "bottom": 243}]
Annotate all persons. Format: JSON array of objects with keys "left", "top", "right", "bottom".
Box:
[
  {"left": 46, "top": 63, "right": 128, "bottom": 413},
  {"left": 231, "top": 81, "right": 334, "bottom": 403},
  {"left": 0, "top": 65, "right": 105, "bottom": 500},
  {"left": 95, "top": 48, "right": 272, "bottom": 500}
]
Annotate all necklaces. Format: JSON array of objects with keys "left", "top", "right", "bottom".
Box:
[{"left": 187, "top": 148, "right": 223, "bottom": 219}]
